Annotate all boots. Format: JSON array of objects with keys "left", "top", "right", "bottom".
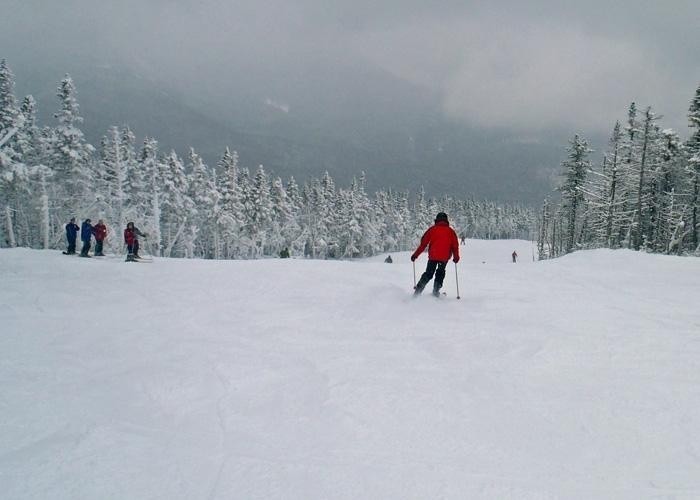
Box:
[{"left": 417, "top": 277, "right": 441, "bottom": 293}]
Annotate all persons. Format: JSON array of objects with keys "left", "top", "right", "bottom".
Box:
[
  {"left": 94, "top": 219, "right": 107, "bottom": 257},
  {"left": 279, "top": 247, "right": 290, "bottom": 258},
  {"left": 66, "top": 218, "right": 80, "bottom": 252},
  {"left": 80, "top": 218, "right": 96, "bottom": 258},
  {"left": 512, "top": 251, "right": 518, "bottom": 263},
  {"left": 461, "top": 236, "right": 466, "bottom": 244},
  {"left": 385, "top": 256, "right": 392, "bottom": 263},
  {"left": 412, "top": 212, "right": 460, "bottom": 296},
  {"left": 123, "top": 220, "right": 146, "bottom": 262}
]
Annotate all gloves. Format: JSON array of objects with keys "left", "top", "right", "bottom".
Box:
[
  {"left": 411, "top": 254, "right": 416, "bottom": 261},
  {"left": 453, "top": 257, "right": 459, "bottom": 263}
]
